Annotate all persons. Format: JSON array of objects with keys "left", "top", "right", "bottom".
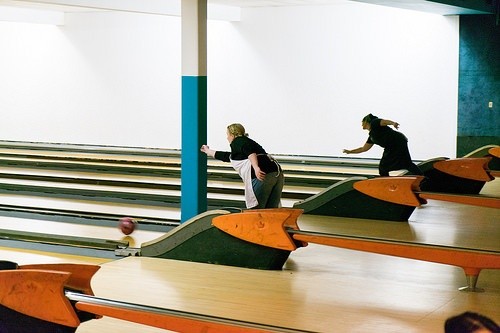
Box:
[
  {"left": 342, "top": 114, "right": 434, "bottom": 186},
  {"left": 200, "top": 124, "right": 284, "bottom": 209}
]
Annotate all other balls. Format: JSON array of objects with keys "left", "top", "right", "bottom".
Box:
[{"left": 120, "top": 219, "right": 135, "bottom": 235}]
